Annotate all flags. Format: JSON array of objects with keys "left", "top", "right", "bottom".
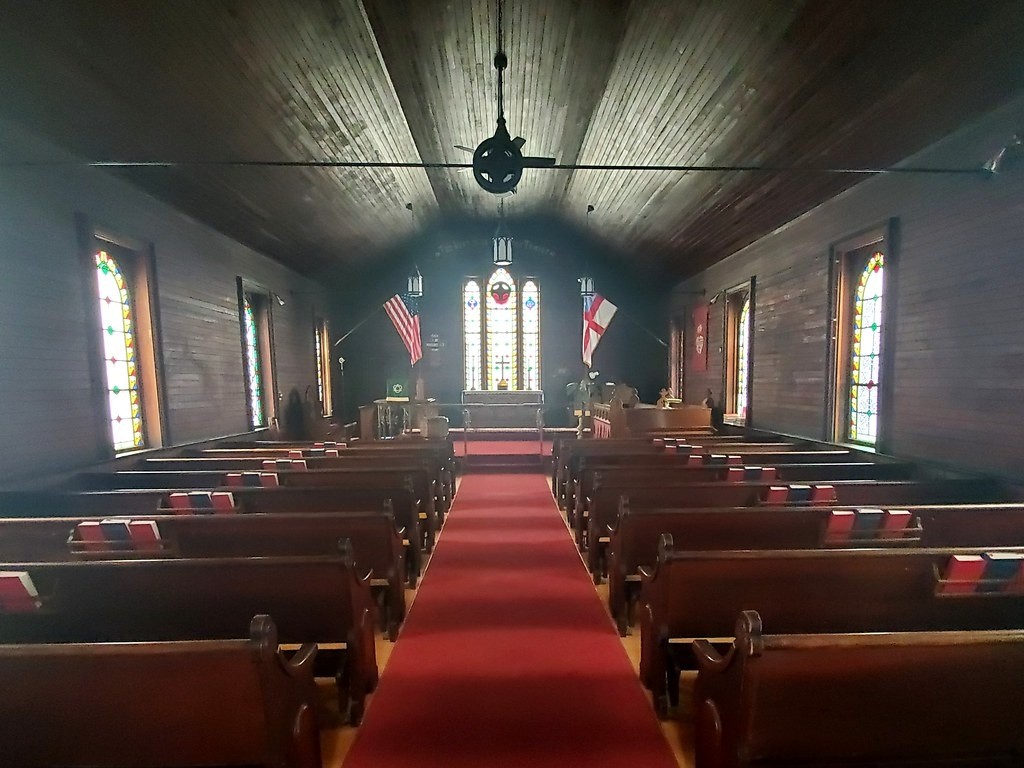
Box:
[
  {"left": 382, "top": 288, "right": 423, "bottom": 368},
  {"left": 583, "top": 293, "right": 618, "bottom": 369}
]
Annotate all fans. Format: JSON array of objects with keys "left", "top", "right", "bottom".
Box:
[{"left": 453, "top": 0, "right": 556, "bottom": 194}]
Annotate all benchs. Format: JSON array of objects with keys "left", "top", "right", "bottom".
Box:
[
  {"left": 0, "top": 418, "right": 458, "bottom": 768},
  {"left": 549, "top": 428, "right": 1024, "bottom": 768}
]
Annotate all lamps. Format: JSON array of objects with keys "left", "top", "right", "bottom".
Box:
[
  {"left": 274, "top": 292, "right": 285, "bottom": 306},
  {"left": 492, "top": 194, "right": 513, "bottom": 267},
  {"left": 579, "top": 205, "right": 596, "bottom": 297},
  {"left": 981, "top": 132, "right": 1022, "bottom": 175},
  {"left": 406, "top": 202, "right": 423, "bottom": 297}
]
